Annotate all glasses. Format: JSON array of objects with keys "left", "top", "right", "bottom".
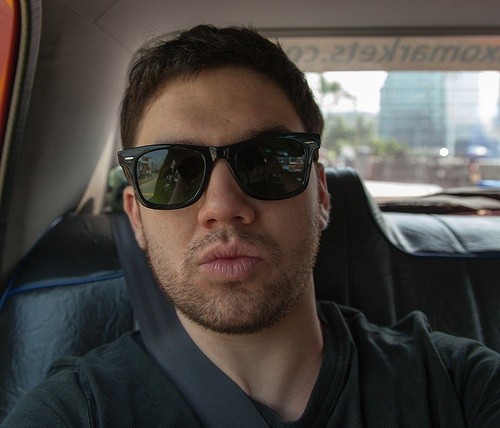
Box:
[{"left": 117, "top": 132, "right": 322, "bottom": 210}]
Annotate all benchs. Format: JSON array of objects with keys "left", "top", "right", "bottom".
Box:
[{"left": 6, "top": 167, "right": 500, "bottom": 428}]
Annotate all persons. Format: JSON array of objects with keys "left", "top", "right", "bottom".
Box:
[{"left": 0, "top": 22, "right": 500, "bottom": 428}]
